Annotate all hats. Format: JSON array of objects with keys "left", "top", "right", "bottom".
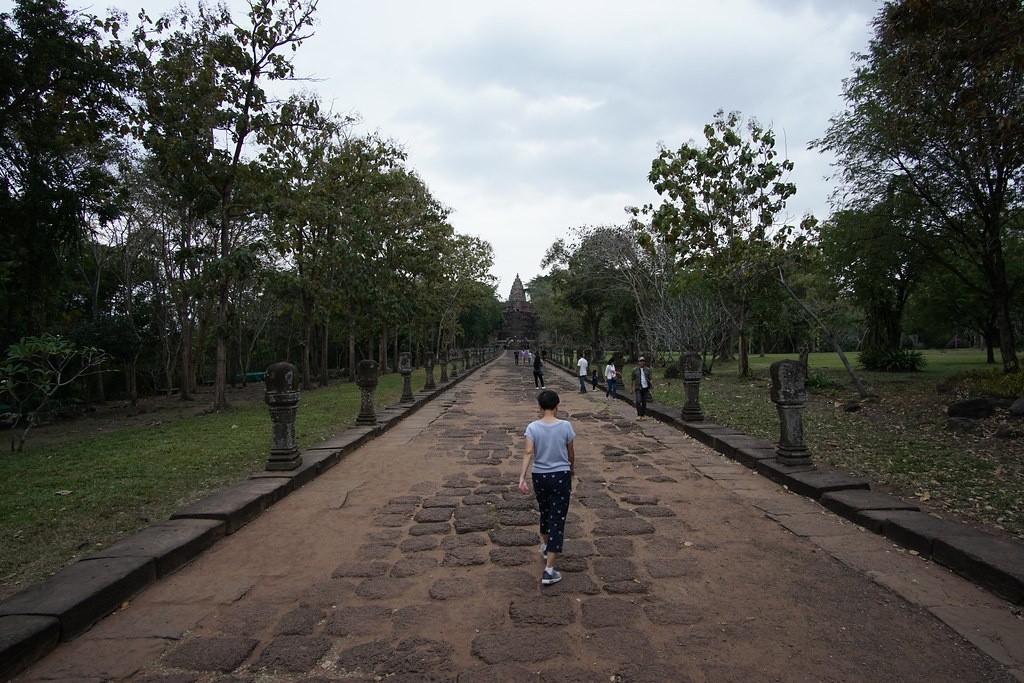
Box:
[{"left": 638, "top": 357, "right": 645, "bottom": 361}]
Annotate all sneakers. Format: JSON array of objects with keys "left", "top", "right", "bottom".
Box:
[
  {"left": 542, "top": 570, "right": 562, "bottom": 584},
  {"left": 541, "top": 543, "right": 547, "bottom": 559}
]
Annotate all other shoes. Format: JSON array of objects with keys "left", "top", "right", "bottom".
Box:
[
  {"left": 542, "top": 386, "right": 546, "bottom": 390},
  {"left": 593, "top": 390, "right": 597, "bottom": 391},
  {"left": 606, "top": 397, "right": 609, "bottom": 400},
  {"left": 535, "top": 387, "right": 539, "bottom": 389},
  {"left": 636, "top": 416, "right": 646, "bottom": 421}
]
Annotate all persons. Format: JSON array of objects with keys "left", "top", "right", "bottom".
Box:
[
  {"left": 591, "top": 366, "right": 600, "bottom": 391},
  {"left": 630, "top": 357, "right": 653, "bottom": 421},
  {"left": 576, "top": 353, "right": 589, "bottom": 394},
  {"left": 605, "top": 358, "right": 621, "bottom": 401},
  {"left": 514, "top": 348, "right": 546, "bottom": 390},
  {"left": 519, "top": 391, "right": 576, "bottom": 584}
]
